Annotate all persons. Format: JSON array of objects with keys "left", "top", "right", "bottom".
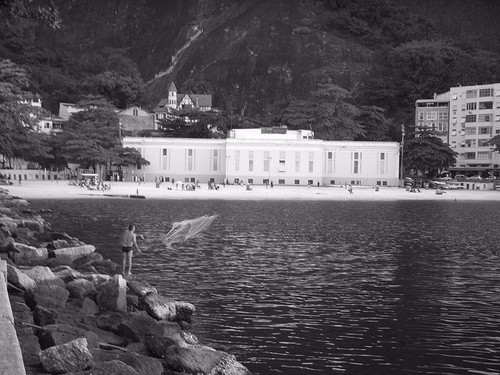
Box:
[
  {"left": 0, "top": 223, "right": 14, "bottom": 252},
  {"left": 69, "top": 172, "right": 276, "bottom": 191},
  {"left": 348, "top": 184, "right": 352, "bottom": 194},
  {"left": 373, "top": 185, "right": 443, "bottom": 195},
  {"left": 121, "top": 224, "right": 142, "bottom": 275}
]
